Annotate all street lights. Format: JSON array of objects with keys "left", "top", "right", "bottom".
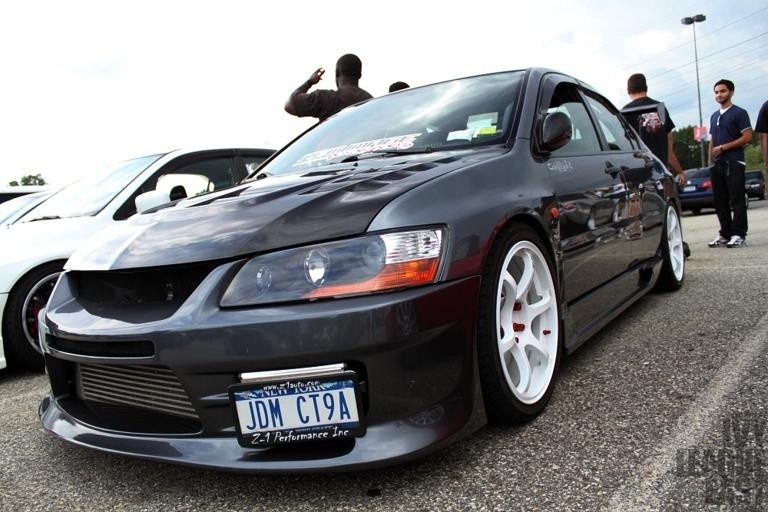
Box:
[{"left": 681, "top": 11, "right": 707, "bottom": 170}]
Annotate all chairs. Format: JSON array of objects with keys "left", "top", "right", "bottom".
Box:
[
  {"left": 170, "top": 185, "right": 187, "bottom": 201},
  {"left": 215, "top": 174, "right": 231, "bottom": 190}
]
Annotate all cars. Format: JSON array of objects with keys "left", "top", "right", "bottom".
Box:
[
  {"left": 744, "top": 170, "right": 766, "bottom": 200},
  {"left": 0, "top": 144, "right": 278, "bottom": 377},
  {"left": 675, "top": 168, "right": 749, "bottom": 214},
  {"left": 28, "top": 62, "right": 692, "bottom": 482}
]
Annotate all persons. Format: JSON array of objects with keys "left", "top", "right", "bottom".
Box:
[
  {"left": 755, "top": 100, "right": 767, "bottom": 200},
  {"left": 282, "top": 52, "right": 376, "bottom": 123},
  {"left": 387, "top": 81, "right": 410, "bottom": 93},
  {"left": 617, "top": 71, "right": 691, "bottom": 259},
  {"left": 705, "top": 77, "right": 753, "bottom": 248}
]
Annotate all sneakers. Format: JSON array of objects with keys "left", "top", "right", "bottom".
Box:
[
  {"left": 708, "top": 236, "right": 729, "bottom": 248},
  {"left": 727, "top": 235, "right": 746, "bottom": 247}
]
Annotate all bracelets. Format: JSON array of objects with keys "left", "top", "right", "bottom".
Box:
[{"left": 719, "top": 145, "right": 723, "bottom": 153}]
